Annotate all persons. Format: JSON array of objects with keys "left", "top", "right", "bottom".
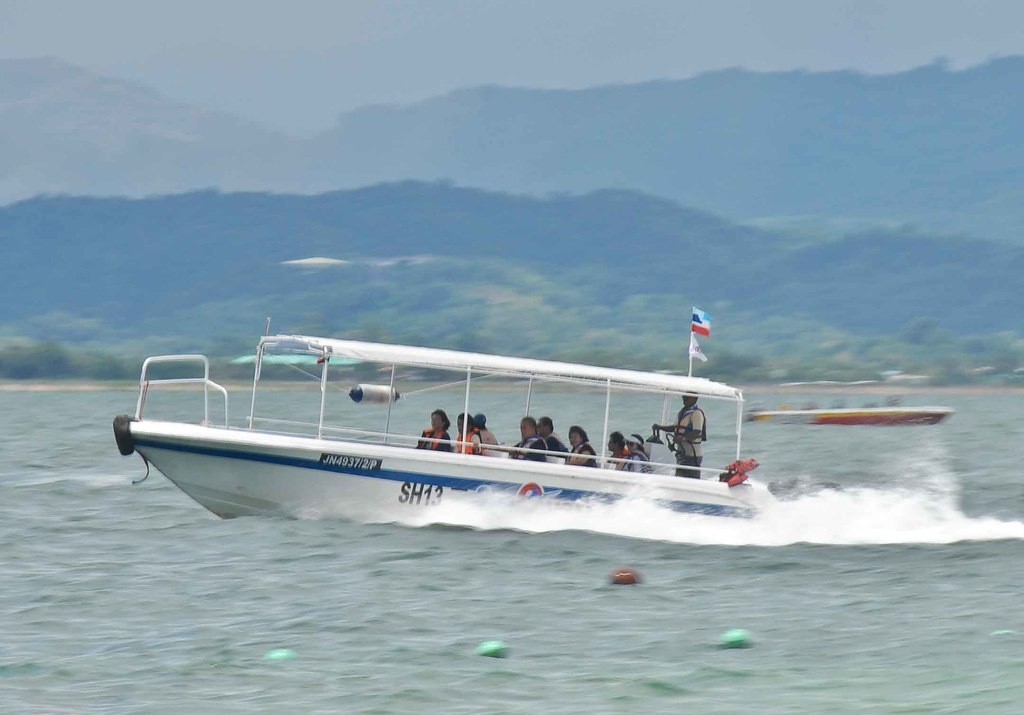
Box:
[
  {"left": 452, "top": 411, "right": 482, "bottom": 455},
  {"left": 563, "top": 425, "right": 597, "bottom": 468},
  {"left": 510, "top": 416, "right": 548, "bottom": 463},
  {"left": 537, "top": 416, "right": 570, "bottom": 459},
  {"left": 606, "top": 431, "right": 629, "bottom": 464},
  {"left": 414, "top": 408, "right": 451, "bottom": 453},
  {"left": 615, "top": 432, "right": 652, "bottom": 473},
  {"left": 653, "top": 390, "right": 708, "bottom": 478},
  {"left": 473, "top": 413, "right": 498, "bottom": 446}
]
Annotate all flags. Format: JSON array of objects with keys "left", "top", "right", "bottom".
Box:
[
  {"left": 689, "top": 332, "right": 708, "bottom": 362},
  {"left": 690, "top": 306, "right": 712, "bottom": 338}
]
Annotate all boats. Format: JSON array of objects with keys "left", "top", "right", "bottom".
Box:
[
  {"left": 742, "top": 398, "right": 954, "bottom": 426},
  {"left": 111, "top": 328, "right": 848, "bottom": 535}
]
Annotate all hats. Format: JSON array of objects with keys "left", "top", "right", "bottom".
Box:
[
  {"left": 472, "top": 414, "right": 488, "bottom": 430},
  {"left": 625, "top": 433, "right": 645, "bottom": 447}
]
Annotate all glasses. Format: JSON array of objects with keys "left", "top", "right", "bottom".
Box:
[{"left": 626, "top": 441, "right": 637, "bottom": 447}]
